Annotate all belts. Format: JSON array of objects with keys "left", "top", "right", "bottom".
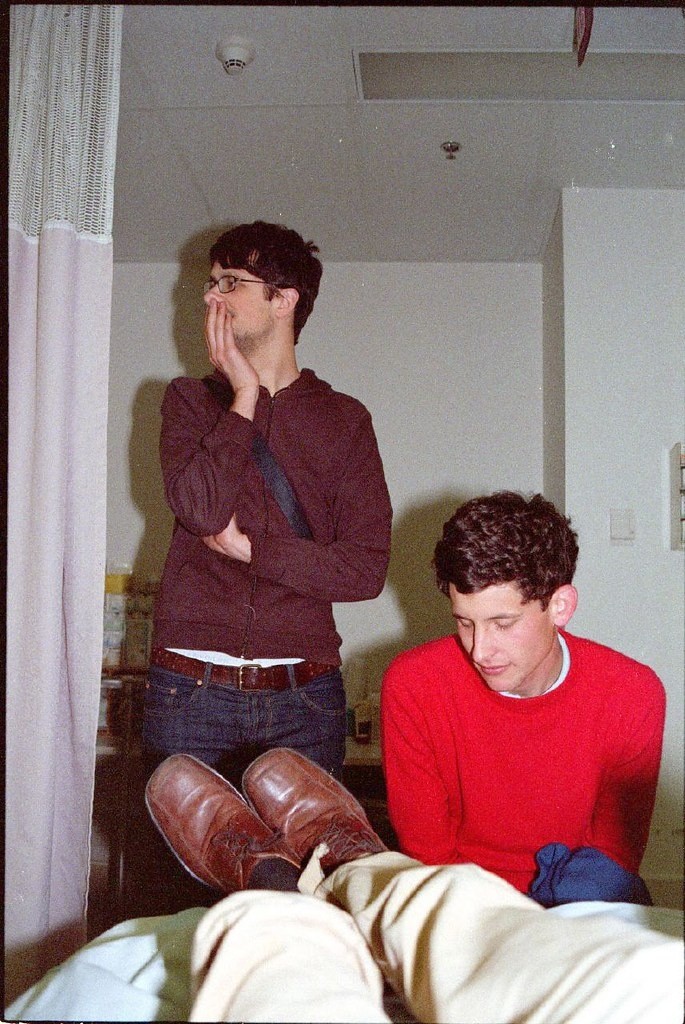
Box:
[{"left": 150, "top": 645, "right": 337, "bottom": 693}]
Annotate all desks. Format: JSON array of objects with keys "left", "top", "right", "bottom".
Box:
[{"left": 96, "top": 734, "right": 382, "bottom": 917}]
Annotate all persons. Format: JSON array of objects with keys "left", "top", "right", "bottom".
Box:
[
  {"left": 145, "top": 224, "right": 394, "bottom": 914},
  {"left": 145, "top": 748, "right": 685, "bottom": 1023},
  {"left": 382, "top": 491, "right": 666, "bottom": 905}
]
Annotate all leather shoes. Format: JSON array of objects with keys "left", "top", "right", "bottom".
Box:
[
  {"left": 143, "top": 751, "right": 307, "bottom": 898},
  {"left": 244, "top": 747, "right": 387, "bottom": 876}
]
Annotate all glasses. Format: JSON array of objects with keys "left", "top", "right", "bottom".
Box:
[{"left": 204, "top": 275, "right": 285, "bottom": 294}]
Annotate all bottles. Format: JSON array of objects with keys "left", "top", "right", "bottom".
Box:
[
  {"left": 125, "top": 582, "right": 159, "bottom": 674},
  {"left": 101, "top": 573, "right": 137, "bottom": 673},
  {"left": 343, "top": 658, "right": 368, "bottom": 738}
]
